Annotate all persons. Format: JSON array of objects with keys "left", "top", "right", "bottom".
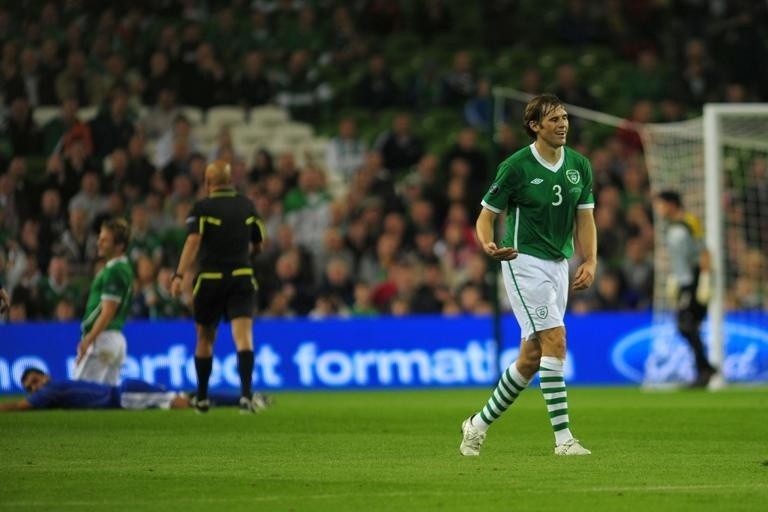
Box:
[
  {"left": 168, "top": 158, "right": 265, "bottom": 415},
  {"left": 0, "top": 365, "right": 276, "bottom": 412},
  {"left": 69, "top": 216, "right": 135, "bottom": 387},
  {"left": 455, "top": 92, "right": 599, "bottom": 457},
  {"left": 650, "top": 190, "right": 724, "bottom": 391},
  {"left": 2, "top": 0, "right": 768, "bottom": 324}
]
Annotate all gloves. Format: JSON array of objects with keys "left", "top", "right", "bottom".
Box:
[
  {"left": 665, "top": 278, "right": 678, "bottom": 299},
  {"left": 694, "top": 270, "right": 712, "bottom": 305}
]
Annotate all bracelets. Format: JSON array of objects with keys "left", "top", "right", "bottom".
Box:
[{"left": 170, "top": 272, "right": 186, "bottom": 281}]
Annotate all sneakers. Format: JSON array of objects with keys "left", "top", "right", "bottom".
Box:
[
  {"left": 191, "top": 395, "right": 210, "bottom": 414},
  {"left": 554, "top": 438, "right": 592, "bottom": 456},
  {"left": 459, "top": 414, "right": 487, "bottom": 456},
  {"left": 239, "top": 395, "right": 252, "bottom": 414}
]
